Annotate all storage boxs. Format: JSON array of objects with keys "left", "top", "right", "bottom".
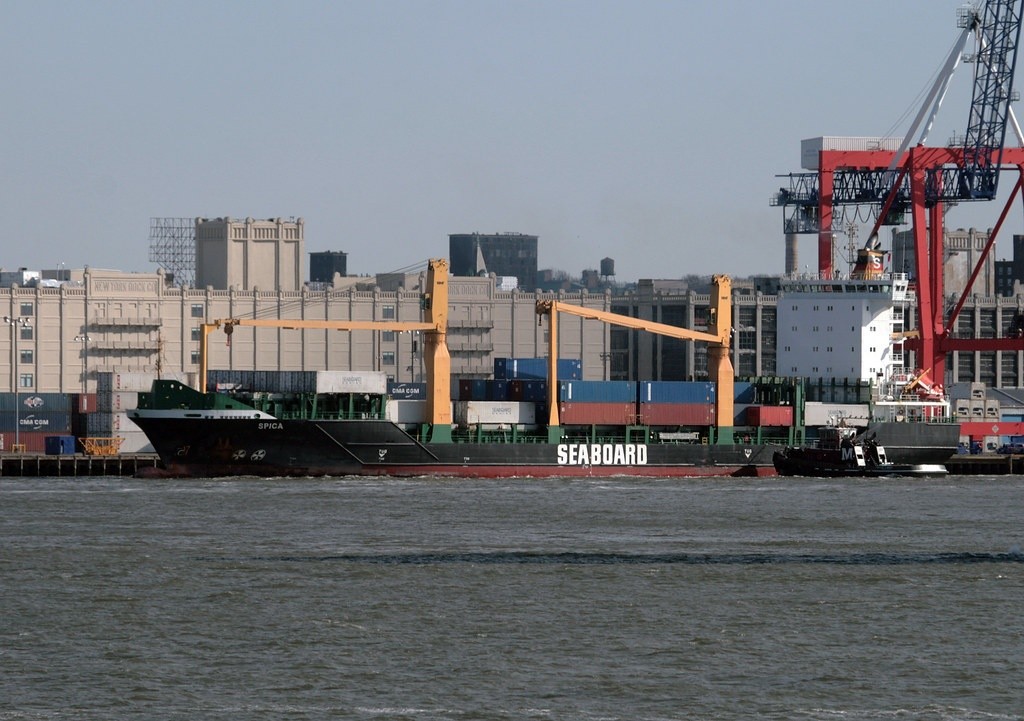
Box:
[
  {"left": 0, "top": 370, "right": 189, "bottom": 456},
  {"left": 209, "top": 358, "right": 878, "bottom": 447}
]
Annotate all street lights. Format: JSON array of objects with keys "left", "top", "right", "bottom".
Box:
[
  {"left": 3, "top": 315, "right": 31, "bottom": 452},
  {"left": 75, "top": 333, "right": 93, "bottom": 438}
]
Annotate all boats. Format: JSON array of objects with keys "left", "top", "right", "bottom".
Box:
[
  {"left": 124, "top": 260, "right": 1023, "bottom": 478},
  {"left": 772, "top": 420, "right": 961, "bottom": 479}
]
